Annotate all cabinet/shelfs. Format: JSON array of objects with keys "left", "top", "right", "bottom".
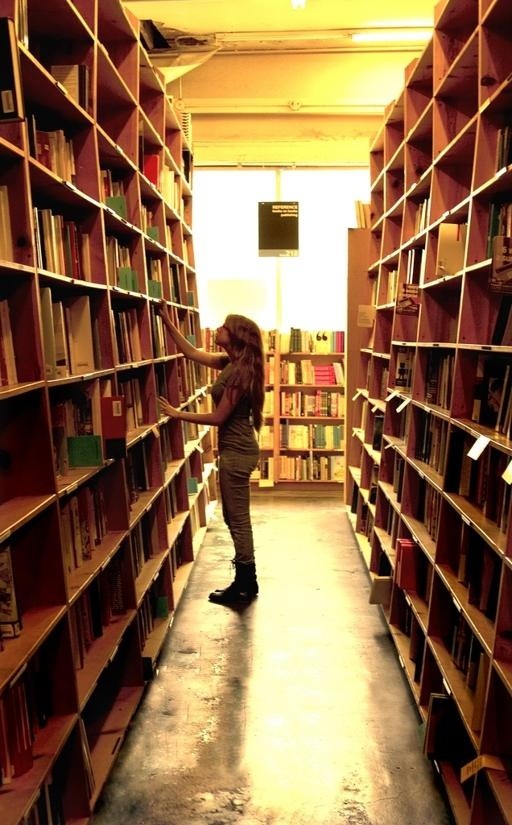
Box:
[
  {"left": 0, "top": 1, "right": 217, "bottom": 825},
  {"left": 341, "top": 0, "right": 512, "bottom": 824},
  {"left": 198, "top": 322, "right": 348, "bottom": 503}
]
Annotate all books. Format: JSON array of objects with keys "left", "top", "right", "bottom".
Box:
[
  {"left": 348, "top": 104, "right": 509, "bottom": 824},
  {"left": 1, "top": 0, "right": 199, "bottom": 825},
  {"left": 199, "top": 327, "right": 346, "bottom": 486}
]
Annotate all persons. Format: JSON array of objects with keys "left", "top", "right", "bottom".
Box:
[{"left": 155, "top": 296, "right": 267, "bottom": 610}]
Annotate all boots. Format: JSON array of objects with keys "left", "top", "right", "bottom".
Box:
[
  {"left": 215, "top": 561, "right": 259, "bottom": 596},
  {"left": 205, "top": 559, "right": 256, "bottom": 605}
]
